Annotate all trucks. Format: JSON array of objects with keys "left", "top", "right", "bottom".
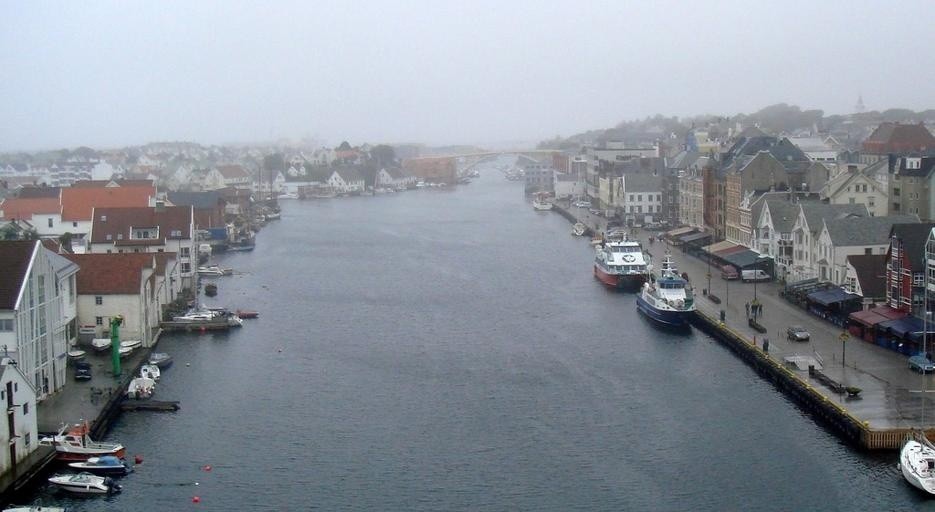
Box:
[{"left": 740, "top": 269, "right": 772, "bottom": 282}]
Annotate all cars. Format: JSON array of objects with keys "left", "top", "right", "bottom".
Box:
[
  {"left": 573, "top": 198, "right": 675, "bottom": 239},
  {"left": 75, "top": 361, "right": 92, "bottom": 380},
  {"left": 787, "top": 324, "right": 811, "bottom": 342},
  {"left": 908, "top": 355, "right": 934, "bottom": 374}
]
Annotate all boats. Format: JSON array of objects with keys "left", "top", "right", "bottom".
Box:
[
  {"left": 173, "top": 198, "right": 282, "bottom": 327},
  {"left": 67, "top": 337, "right": 141, "bottom": 364},
  {"left": 634, "top": 245, "right": 698, "bottom": 329},
  {"left": 594, "top": 229, "right": 653, "bottom": 292},
  {"left": 126, "top": 351, "right": 175, "bottom": 401},
  {"left": 37, "top": 417, "right": 127, "bottom": 462},
  {"left": 504, "top": 165, "right": 525, "bottom": 182},
  {"left": 1, "top": 499, "right": 70, "bottom": 512},
  {"left": 65, "top": 457, "right": 134, "bottom": 476},
  {"left": 532, "top": 189, "right": 555, "bottom": 211},
  {"left": 47, "top": 473, "right": 124, "bottom": 495}
]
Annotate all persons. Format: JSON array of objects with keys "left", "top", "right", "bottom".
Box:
[
  {"left": 744, "top": 300, "right": 751, "bottom": 320},
  {"left": 758, "top": 304, "right": 763, "bottom": 317}
]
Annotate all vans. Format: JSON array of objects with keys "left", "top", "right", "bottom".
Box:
[{"left": 722, "top": 265, "right": 738, "bottom": 280}]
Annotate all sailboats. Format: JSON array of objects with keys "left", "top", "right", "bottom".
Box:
[{"left": 897, "top": 253, "right": 935, "bottom": 494}]
